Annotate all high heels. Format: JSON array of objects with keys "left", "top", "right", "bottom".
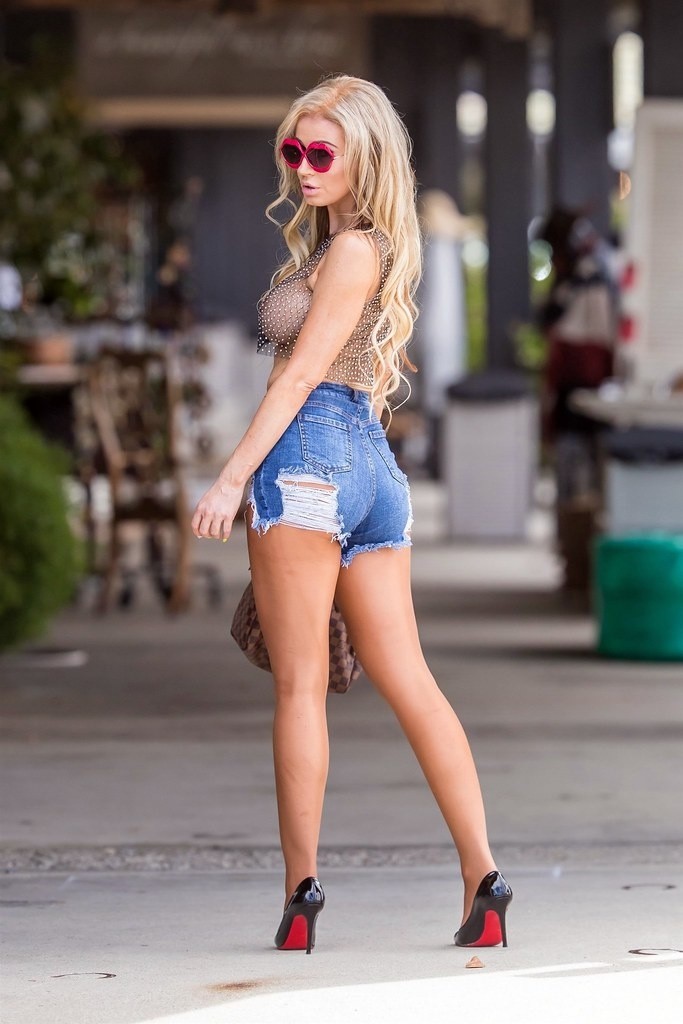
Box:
[
  {"left": 454, "top": 870, "right": 512, "bottom": 947},
  {"left": 274, "top": 877, "right": 325, "bottom": 955}
]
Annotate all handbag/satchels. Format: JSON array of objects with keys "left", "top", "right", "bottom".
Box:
[{"left": 231, "top": 572, "right": 365, "bottom": 695}]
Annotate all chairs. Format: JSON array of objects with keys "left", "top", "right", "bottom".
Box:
[{"left": 16, "top": 337, "right": 223, "bottom": 613}]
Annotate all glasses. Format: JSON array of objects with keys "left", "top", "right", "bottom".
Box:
[{"left": 281, "top": 136, "right": 346, "bottom": 173}]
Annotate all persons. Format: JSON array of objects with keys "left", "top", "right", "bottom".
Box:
[{"left": 191, "top": 77, "right": 512, "bottom": 953}]
